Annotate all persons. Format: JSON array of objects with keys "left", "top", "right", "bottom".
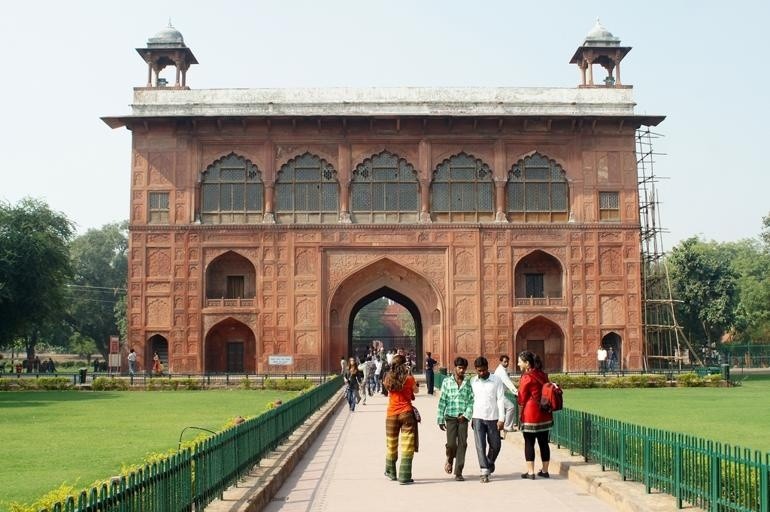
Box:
[
  {"left": 493, "top": 354, "right": 520, "bottom": 439},
  {"left": 150, "top": 351, "right": 160, "bottom": 375},
  {"left": 339, "top": 344, "right": 439, "bottom": 412},
  {"left": 607, "top": 346, "right": 619, "bottom": 370},
  {"left": 32, "top": 354, "right": 109, "bottom": 376},
  {"left": 515, "top": 349, "right": 555, "bottom": 480},
  {"left": 159, "top": 360, "right": 166, "bottom": 377},
  {"left": 381, "top": 354, "right": 420, "bottom": 485},
  {"left": 0, "top": 353, "right": 5, "bottom": 390},
  {"left": 436, "top": 356, "right": 475, "bottom": 482},
  {"left": 127, "top": 348, "right": 137, "bottom": 376},
  {"left": 469, "top": 357, "right": 506, "bottom": 483},
  {"left": 597, "top": 345, "right": 608, "bottom": 373}
]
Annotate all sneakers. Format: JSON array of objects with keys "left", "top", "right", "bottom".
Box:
[
  {"left": 537, "top": 470, "right": 550, "bottom": 478},
  {"left": 479, "top": 473, "right": 490, "bottom": 483},
  {"left": 521, "top": 472, "right": 535, "bottom": 480},
  {"left": 399, "top": 478, "right": 414, "bottom": 485},
  {"left": 444, "top": 459, "right": 464, "bottom": 481}
]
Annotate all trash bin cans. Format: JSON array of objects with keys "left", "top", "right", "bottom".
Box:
[
  {"left": 78, "top": 369, "right": 87, "bottom": 383},
  {"left": 721, "top": 364, "right": 729, "bottom": 380}
]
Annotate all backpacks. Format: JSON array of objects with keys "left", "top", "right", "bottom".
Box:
[{"left": 524, "top": 371, "right": 563, "bottom": 413}]
